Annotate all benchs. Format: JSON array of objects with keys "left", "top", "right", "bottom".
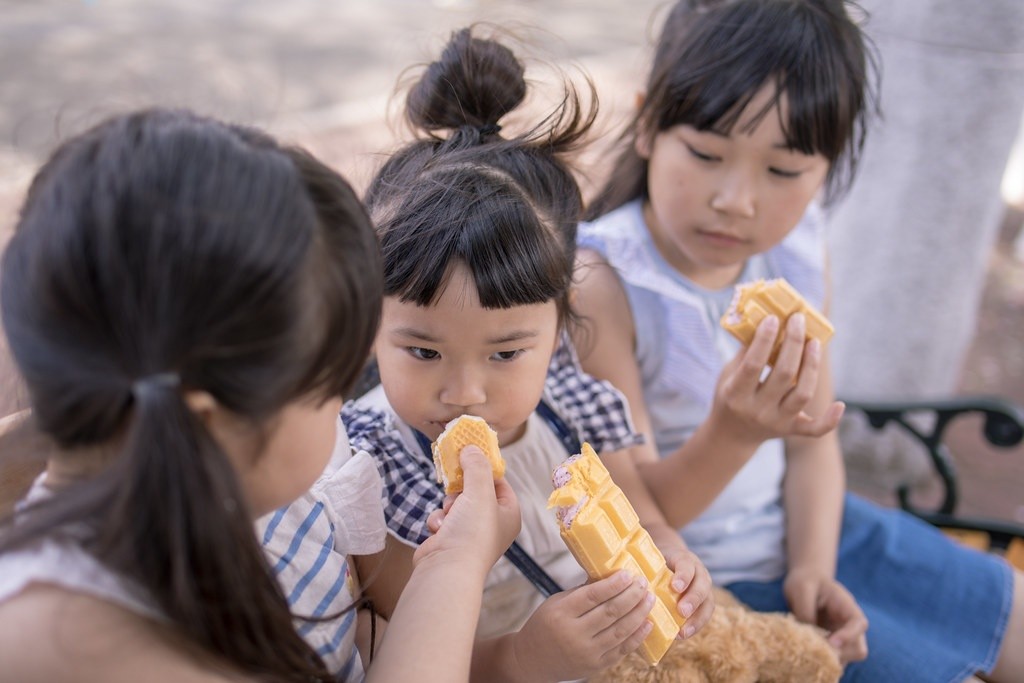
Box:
[{"left": 838, "top": 398, "right": 1024, "bottom": 557}]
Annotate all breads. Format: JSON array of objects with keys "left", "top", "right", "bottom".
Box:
[
  {"left": 719, "top": 276, "right": 835, "bottom": 383},
  {"left": 546, "top": 441, "right": 687, "bottom": 668},
  {"left": 431, "top": 413, "right": 505, "bottom": 495}
]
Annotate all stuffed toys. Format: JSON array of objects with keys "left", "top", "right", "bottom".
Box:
[{"left": 580, "top": 575, "right": 843, "bottom": 683}]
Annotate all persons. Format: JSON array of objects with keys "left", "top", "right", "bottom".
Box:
[
  {"left": 563, "top": 0, "right": 1023, "bottom": 683},
  {"left": 0, "top": 105, "right": 521, "bottom": 683},
  {"left": 331, "top": 38, "right": 855, "bottom": 683}
]
